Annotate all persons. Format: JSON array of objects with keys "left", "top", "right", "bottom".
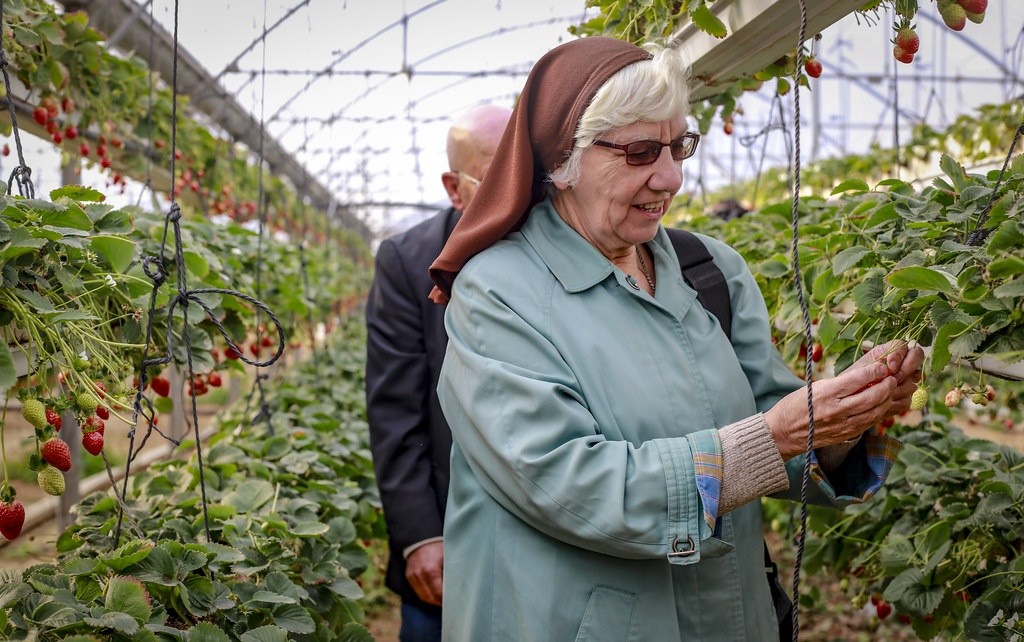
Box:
[
  {"left": 363, "top": 106, "right": 799, "bottom": 642},
  {"left": 427, "top": 35, "right": 924, "bottom": 642}
]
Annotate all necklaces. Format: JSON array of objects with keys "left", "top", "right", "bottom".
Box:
[{"left": 636, "top": 244, "right": 654, "bottom": 297}]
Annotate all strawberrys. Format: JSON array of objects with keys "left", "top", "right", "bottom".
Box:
[
  {"left": 0, "top": 58, "right": 372, "bottom": 541},
  {"left": 725, "top": 0, "right": 1015, "bottom": 628}
]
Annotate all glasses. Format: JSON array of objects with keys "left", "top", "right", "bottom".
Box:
[{"left": 592, "top": 131, "right": 700, "bottom": 166}]
